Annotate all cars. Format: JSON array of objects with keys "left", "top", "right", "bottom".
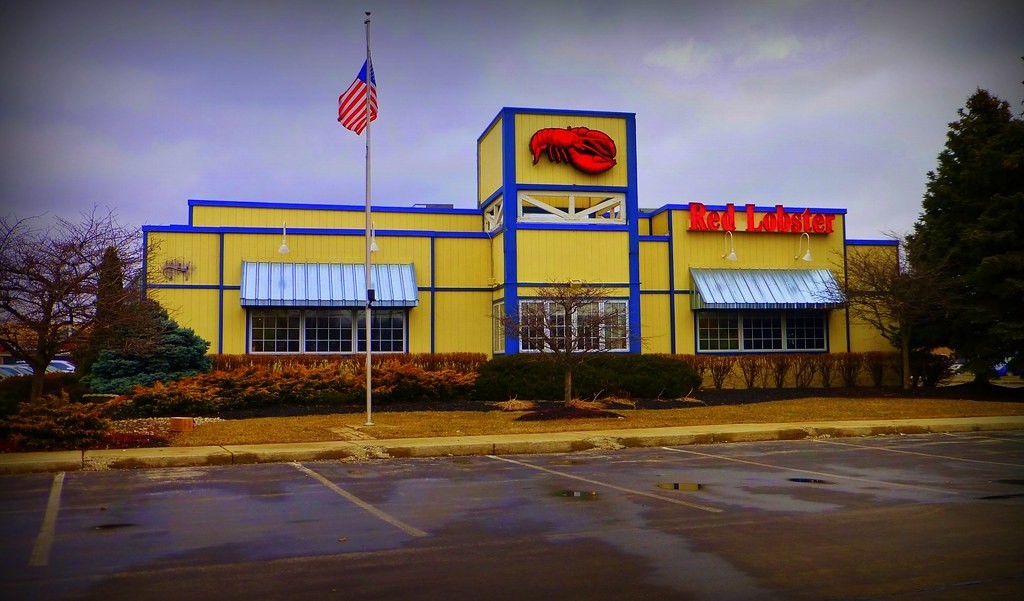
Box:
[
  {"left": 0, "top": 359, "right": 75, "bottom": 385},
  {"left": 937, "top": 354, "right": 964, "bottom": 375}
]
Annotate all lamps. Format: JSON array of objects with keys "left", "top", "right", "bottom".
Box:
[
  {"left": 722, "top": 230, "right": 738, "bottom": 261},
  {"left": 795, "top": 233, "right": 812, "bottom": 263},
  {"left": 370, "top": 220, "right": 380, "bottom": 253},
  {"left": 278, "top": 220, "right": 289, "bottom": 253}
]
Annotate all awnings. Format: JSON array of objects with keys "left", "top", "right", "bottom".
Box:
[
  {"left": 239, "top": 260, "right": 420, "bottom": 310},
  {"left": 687, "top": 267, "right": 849, "bottom": 310}
]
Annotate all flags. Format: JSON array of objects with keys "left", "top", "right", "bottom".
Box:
[{"left": 337, "top": 46, "right": 378, "bottom": 135}]
